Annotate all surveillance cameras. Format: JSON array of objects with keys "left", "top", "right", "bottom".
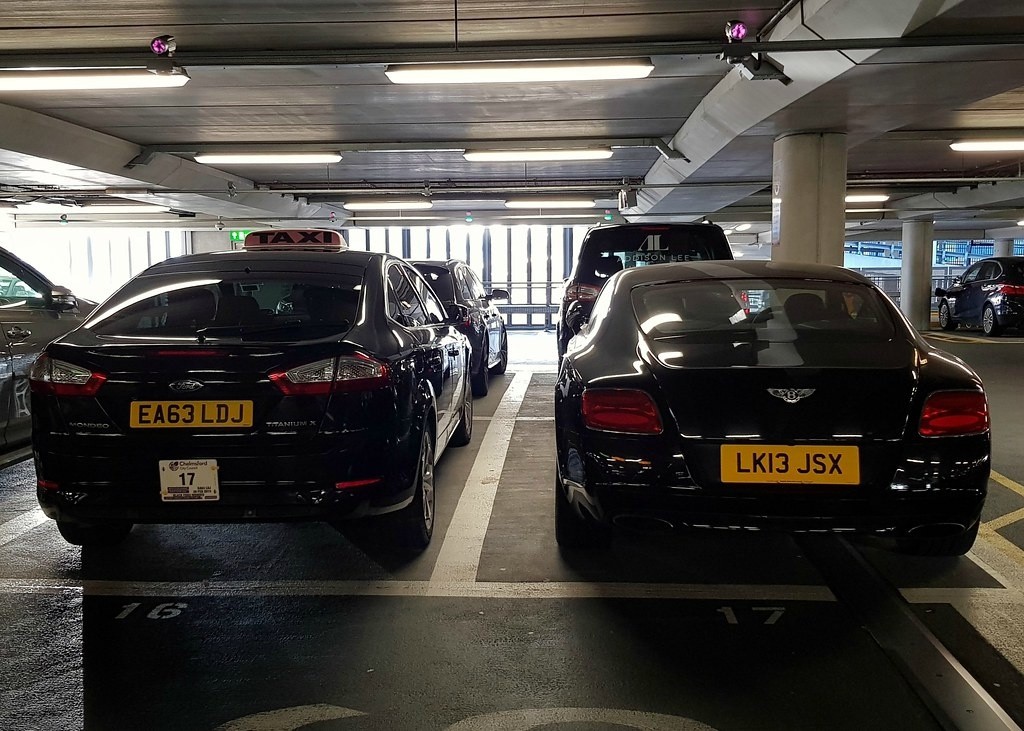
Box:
[
  {"left": 214, "top": 223, "right": 225, "bottom": 230},
  {"left": 150, "top": 35, "right": 176, "bottom": 55},
  {"left": 725, "top": 20, "right": 747, "bottom": 40}
]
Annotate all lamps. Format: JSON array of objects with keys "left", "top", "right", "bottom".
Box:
[
  {"left": 193, "top": 149, "right": 345, "bottom": 165},
  {"left": 949, "top": 138, "right": 1024, "bottom": 153},
  {"left": 343, "top": 200, "right": 435, "bottom": 211},
  {"left": 0, "top": 68, "right": 192, "bottom": 91},
  {"left": 463, "top": 148, "right": 614, "bottom": 163},
  {"left": 505, "top": 199, "right": 597, "bottom": 209},
  {"left": 382, "top": 55, "right": 657, "bottom": 86},
  {"left": 845, "top": 193, "right": 891, "bottom": 203}
]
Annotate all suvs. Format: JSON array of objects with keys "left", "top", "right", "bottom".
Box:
[{"left": 556, "top": 218, "right": 751, "bottom": 373}]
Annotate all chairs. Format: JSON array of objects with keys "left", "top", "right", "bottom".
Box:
[
  {"left": 178, "top": 289, "right": 216, "bottom": 322},
  {"left": 784, "top": 293, "right": 824, "bottom": 325}
]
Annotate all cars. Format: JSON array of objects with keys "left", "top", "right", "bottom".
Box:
[
  {"left": 0, "top": 275, "right": 42, "bottom": 298},
  {"left": 404, "top": 258, "right": 512, "bottom": 399},
  {"left": 29, "top": 229, "right": 474, "bottom": 555},
  {"left": 552, "top": 257, "right": 990, "bottom": 580},
  {"left": 934, "top": 255, "right": 1024, "bottom": 336},
  {"left": 0, "top": 245, "right": 100, "bottom": 437}
]
